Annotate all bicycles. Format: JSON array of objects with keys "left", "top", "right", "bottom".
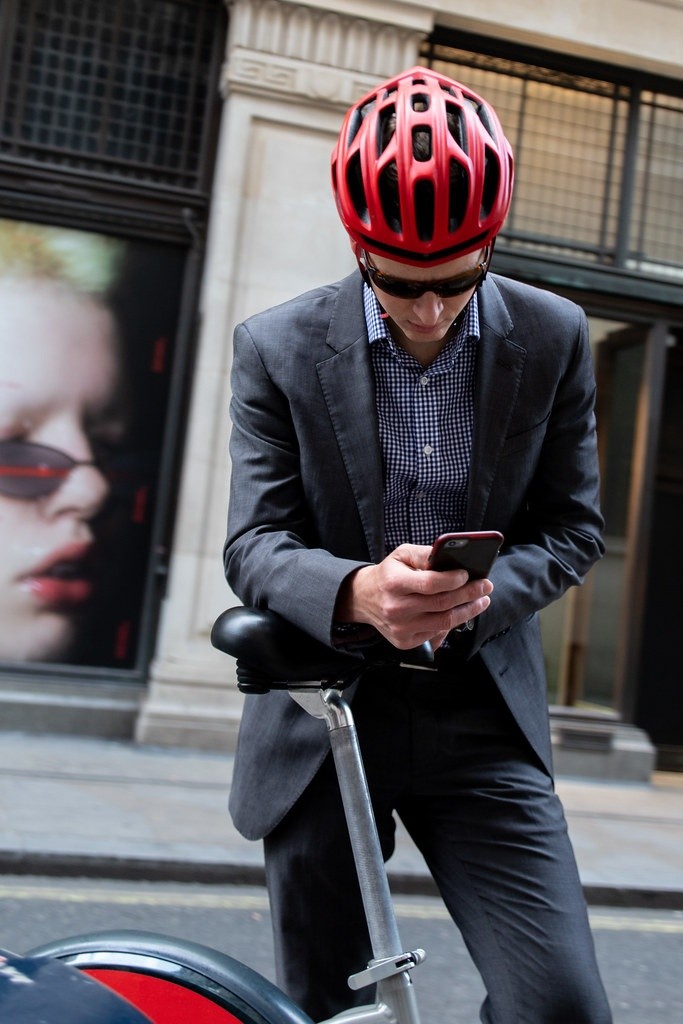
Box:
[{"left": 13, "top": 602, "right": 448, "bottom": 1023}]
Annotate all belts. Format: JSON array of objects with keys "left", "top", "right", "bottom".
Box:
[{"left": 367, "top": 637, "right": 480, "bottom": 671}]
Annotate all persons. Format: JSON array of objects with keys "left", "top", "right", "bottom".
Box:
[
  {"left": 0, "top": 218, "right": 177, "bottom": 671},
  {"left": 219, "top": 69, "right": 616, "bottom": 1024}
]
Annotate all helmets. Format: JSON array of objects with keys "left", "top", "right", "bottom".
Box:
[{"left": 332, "top": 74, "right": 517, "bottom": 266}]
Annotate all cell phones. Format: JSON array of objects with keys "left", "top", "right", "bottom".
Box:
[{"left": 426, "top": 532, "right": 504, "bottom": 581}]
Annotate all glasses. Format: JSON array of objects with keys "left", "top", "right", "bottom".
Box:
[
  {"left": 1, "top": 437, "right": 126, "bottom": 499},
  {"left": 360, "top": 236, "right": 493, "bottom": 295}
]
